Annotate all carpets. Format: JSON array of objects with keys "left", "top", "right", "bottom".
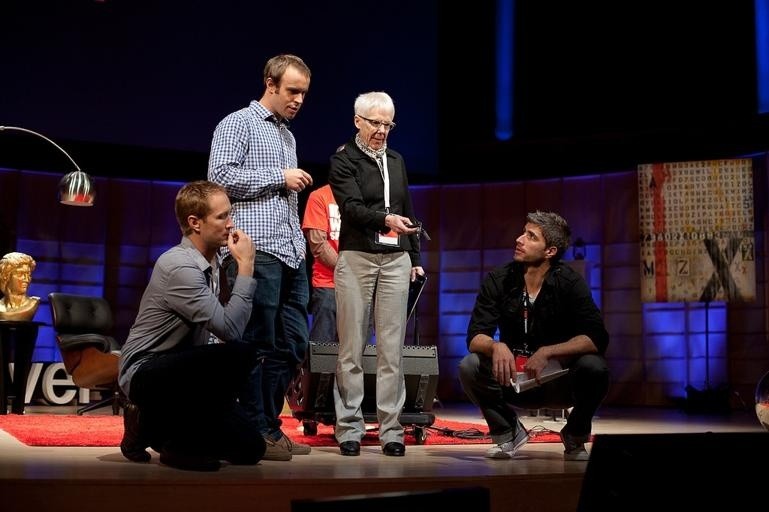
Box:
[{"left": 0, "top": 410, "right": 596, "bottom": 446}]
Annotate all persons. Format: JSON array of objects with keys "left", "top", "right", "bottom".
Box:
[
  {"left": 207, "top": 54, "right": 314, "bottom": 461},
  {"left": 0, "top": 251, "right": 40, "bottom": 322},
  {"left": 457, "top": 209, "right": 610, "bottom": 461},
  {"left": 300, "top": 144, "right": 374, "bottom": 435},
  {"left": 118, "top": 180, "right": 267, "bottom": 472},
  {"left": 328, "top": 90, "right": 425, "bottom": 456}
]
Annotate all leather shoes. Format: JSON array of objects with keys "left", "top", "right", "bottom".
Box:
[
  {"left": 340, "top": 441, "right": 360, "bottom": 456},
  {"left": 383, "top": 442, "right": 405, "bottom": 456},
  {"left": 121, "top": 404, "right": 151, "bottom": 462}
]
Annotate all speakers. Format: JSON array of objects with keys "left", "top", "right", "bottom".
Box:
[{"left": 284, "top": 342, "right": 440, "bottom": 425}]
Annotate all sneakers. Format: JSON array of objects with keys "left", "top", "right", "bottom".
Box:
[
  {"left": 487, "top": 429, "right": 529, "bottom": 458},
  {"left": 263, "top": 434, "right": 311, "bottom": 460},
  {"left": 560, "top": 430, "right": 588, "bottom": 461}
]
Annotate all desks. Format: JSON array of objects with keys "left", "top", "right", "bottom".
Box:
[{"left": 0, "top": 317, "right": 45, "bottom": 413}]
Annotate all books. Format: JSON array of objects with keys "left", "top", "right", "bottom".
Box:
[
  {"left": 406, "top": 272, "right": 427, "bottom": 324},
  {"left": 510, "top": 359, "right": 570, "bottom": 394}
]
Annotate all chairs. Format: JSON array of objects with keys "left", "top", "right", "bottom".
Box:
[
  {"left": 47, "top": 292, "right": 129, "bottom": 416},
  {"left": 285, "top": 482, "right": 495, "bottom": 511}
]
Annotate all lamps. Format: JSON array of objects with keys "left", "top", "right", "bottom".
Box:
[{"left": 1, "top": 123, "right": 96, "bottom": 208}]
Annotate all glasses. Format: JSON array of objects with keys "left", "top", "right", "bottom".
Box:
[{"left": 358, "top": 115, "right": 396, "bottom": 130}]
